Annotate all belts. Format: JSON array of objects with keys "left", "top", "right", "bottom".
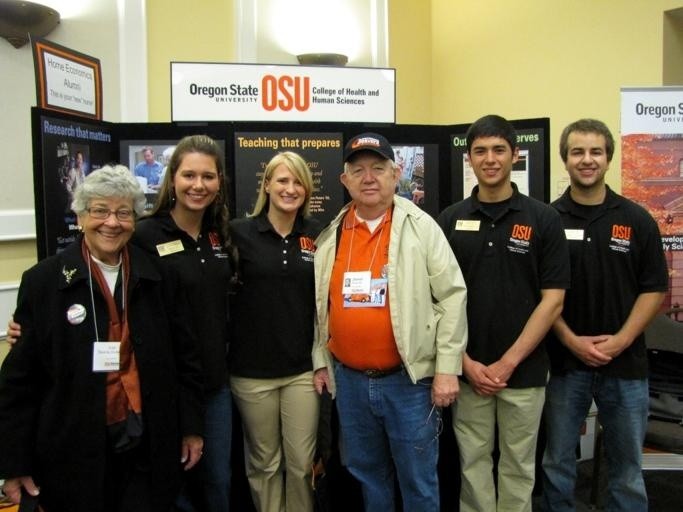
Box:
[{"left": 335, "top": 358, "right": 405, "bottom": 378}]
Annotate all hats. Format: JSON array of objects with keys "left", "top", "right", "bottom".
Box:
[{"left": 342, "top": 133, "right": 395, "bottom": 164}]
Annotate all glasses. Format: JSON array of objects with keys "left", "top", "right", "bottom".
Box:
[{"left": 86, "top": 206, "right": 136, "bottom": 222}]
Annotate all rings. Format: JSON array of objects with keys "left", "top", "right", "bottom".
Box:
[
  {"left": 449, "top": 396, "right": 455, "bottom": 400},
  {"left": 198, "top": 450, "right": 203, "bottom": 454}
]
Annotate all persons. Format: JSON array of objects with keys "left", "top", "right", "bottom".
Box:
[
  {"left": 227, "top": 151, "right": 325, "bottom": 511},
  {"left": 0, "top": 163, "right": 205, "bottom": 512},
  {"left": 540, "top": 118, "right": 668, "bottom": 512},
  {"left": 134, "top": 147, "right": 163, "bottom": 187},
  {"left": 311, "top": 132, "right": 469, "bottom": 512},
  {"left": 434, "top": 115, "right": 572, "bottom": 512},
  {"left": 6, "top": 133, "right": 240, "bottom": 511},
  {"left": 66, "top": 151, "right": 85, "bottom": 207}
]
